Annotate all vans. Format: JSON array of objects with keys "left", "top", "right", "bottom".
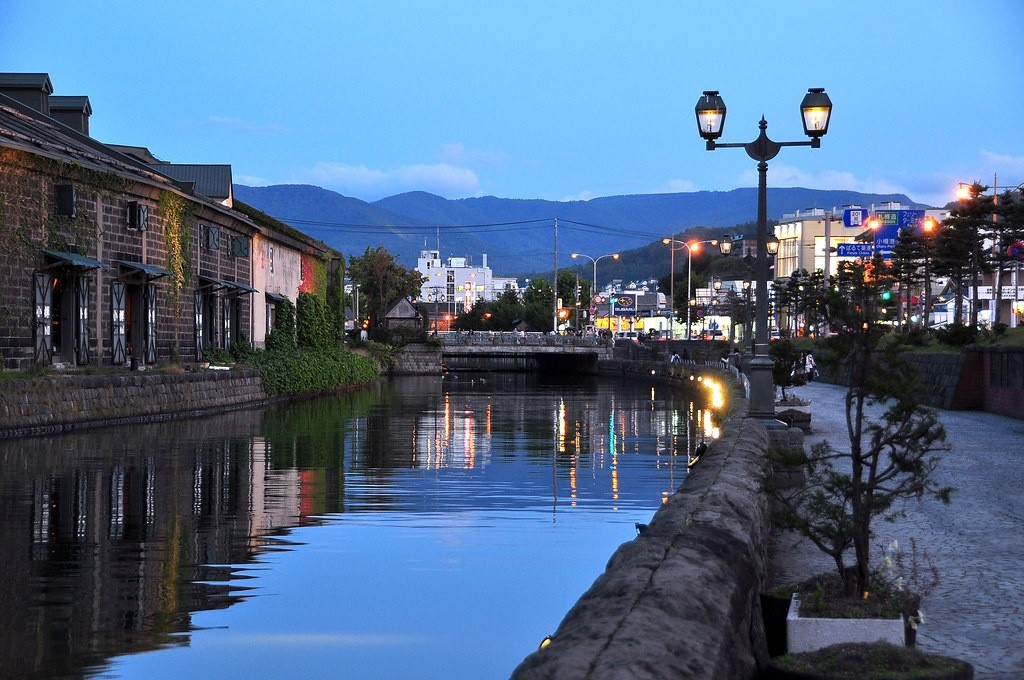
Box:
[{"left": 544, "top": 321, "right": 670, "bottom": 344}]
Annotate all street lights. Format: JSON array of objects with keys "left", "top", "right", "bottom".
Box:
[
  {"left": 570, "top": 253, "right": 620, "bottom": 294},
  {"left": 720, "top": 232, "right": 780, "bottom": 357},
  {"left": 955, "top": 173, "right": 1024, "bottom": 329},
  {"left": 694, "top": 88, "right": 833, "bottom": 431},
  {"left": 427, "top": 289, "right": 445, "bottom": 335},
  {"left": 866, "top": 216, "right": 882, "bottom": 287},
  {"left": 663, "top": 239, "right": 718, "bottom": 340}
]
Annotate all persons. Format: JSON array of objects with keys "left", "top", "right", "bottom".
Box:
[
  {"left": 721, "top": 348, "right": 741, "bottom": 372},
  {"left": 682, "top": 348, "right": 689, "bottom": 361},
  {"left": 452, "top": 327, "right": 660, "bottom": 346},
  {"left": 796, "top": 350, "right": 817, "bottom": 385},
  {"left": 670, "top": 350, "right": 680, "bottom": 362}
]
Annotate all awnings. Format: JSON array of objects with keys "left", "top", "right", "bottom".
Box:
[
  {"left": 41, "top": 249, "right": 109, "bottom": 270},
  {"left": 112, "top": 260, "right": 173, "bottom": 276},
  {"left": 265, "top": 291, "right": 285, "bottom": 303},
  {"left": 198, "top": 274, "right": 260, "bottom": 293}
]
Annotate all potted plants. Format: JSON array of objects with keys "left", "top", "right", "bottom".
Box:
[{"left": 751, "top": 260, "right": 973, "bottom": 680}]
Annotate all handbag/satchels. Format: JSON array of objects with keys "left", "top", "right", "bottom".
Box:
[{"left": 814, "top": 369, "right": 819, "bottom": 377}]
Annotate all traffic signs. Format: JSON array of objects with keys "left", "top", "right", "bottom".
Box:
[{"left": 874, "top": 209, "right": 925, "bottom": 259}]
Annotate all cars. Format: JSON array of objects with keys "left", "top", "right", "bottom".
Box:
[
  {"left": 767, "top": 330, "right": 784, "bottom": 341},
  {"left": 697, "top": 329, "right": 727, "bottom": 342},
  {"left": 344, "top": 284, "right": 354, "bottom": 294}
]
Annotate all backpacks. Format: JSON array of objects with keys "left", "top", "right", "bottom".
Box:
[{"left": 673, "top": 355, "right": 679, "bottom": 362}]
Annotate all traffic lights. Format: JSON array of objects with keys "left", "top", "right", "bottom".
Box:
[
  {"left": 837, "top": 242, "right": 874, "bottom": 257},
  {"left": 609, "top": 292, "right": 615, "bottom": 305},
  {"left": 879, "top": 283, "right": 891, "bottom": 302}
]
